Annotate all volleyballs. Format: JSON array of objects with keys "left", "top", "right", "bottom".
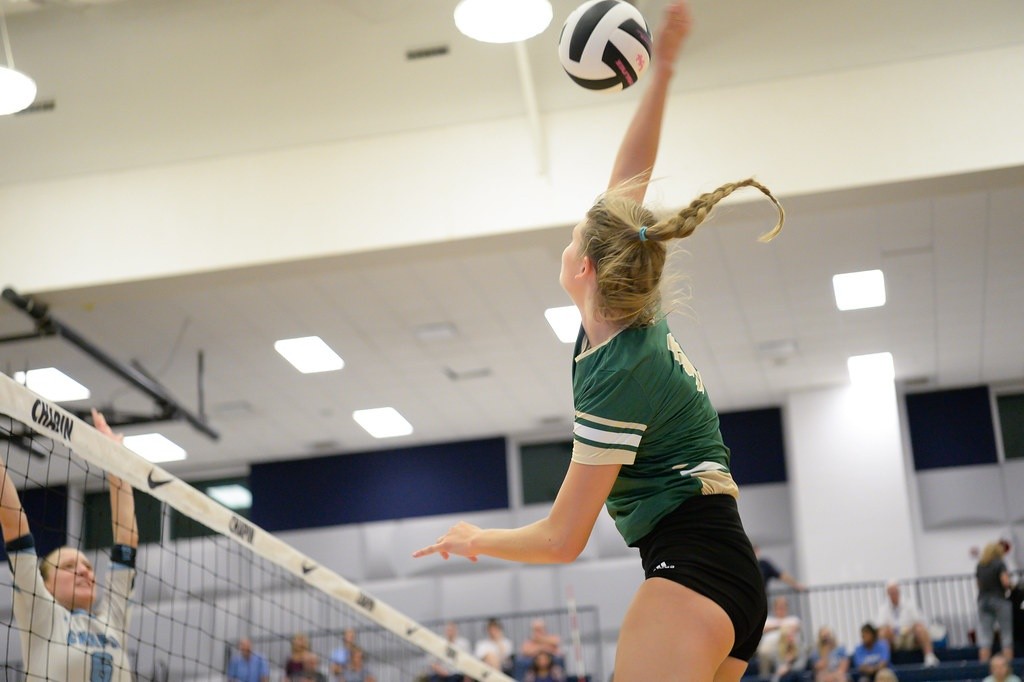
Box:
[{"left": 557, "top": 0, "right": 655, "bottom": 95}]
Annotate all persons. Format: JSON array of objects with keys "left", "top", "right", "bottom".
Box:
[
  {"left": 412, "top": 0, "right": 786, "bottom": 682},
  {"left": 0, "top": 401, "right": 1024, "bottom": 682}
]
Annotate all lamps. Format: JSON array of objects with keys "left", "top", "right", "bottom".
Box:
[
  {"left": 454, "top": 0, "right": 554, "bottom": 44},
  {"left": 0, "top": 8, "right": 37, "bottom": 115}
]
